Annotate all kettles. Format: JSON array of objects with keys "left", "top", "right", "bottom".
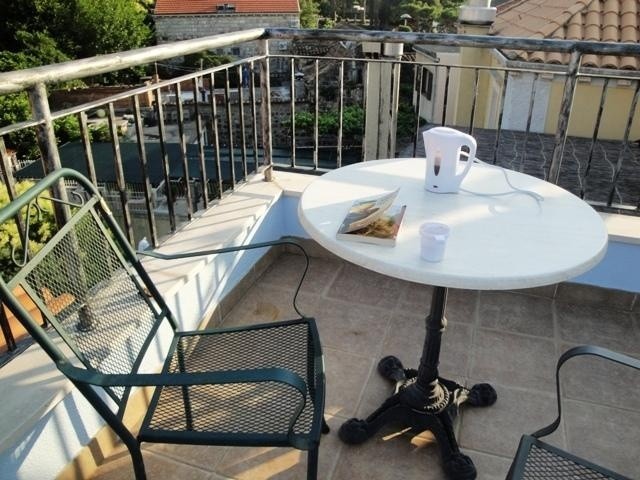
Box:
[{"left": 420, "top": 127, "right": 477, "bottom": 194}]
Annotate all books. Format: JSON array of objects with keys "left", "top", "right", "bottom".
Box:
[{"left": 335, "top": 187, "right": 407, "bottom": 249}]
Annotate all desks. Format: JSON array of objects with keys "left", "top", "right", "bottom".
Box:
[{"left": 296, "top": 157, "right": 610, "bottom": 475}]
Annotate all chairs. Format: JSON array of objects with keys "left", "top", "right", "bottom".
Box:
[
  {"left": 0, "top": 167, "right": 331, "bottom": 480},
  {"left": 506, "top": 338, "right": 640, "bottom": 480}
]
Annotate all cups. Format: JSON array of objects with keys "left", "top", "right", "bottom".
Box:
[{"left": 418, "top": 222, "right": 450, "bottom": 262}]
